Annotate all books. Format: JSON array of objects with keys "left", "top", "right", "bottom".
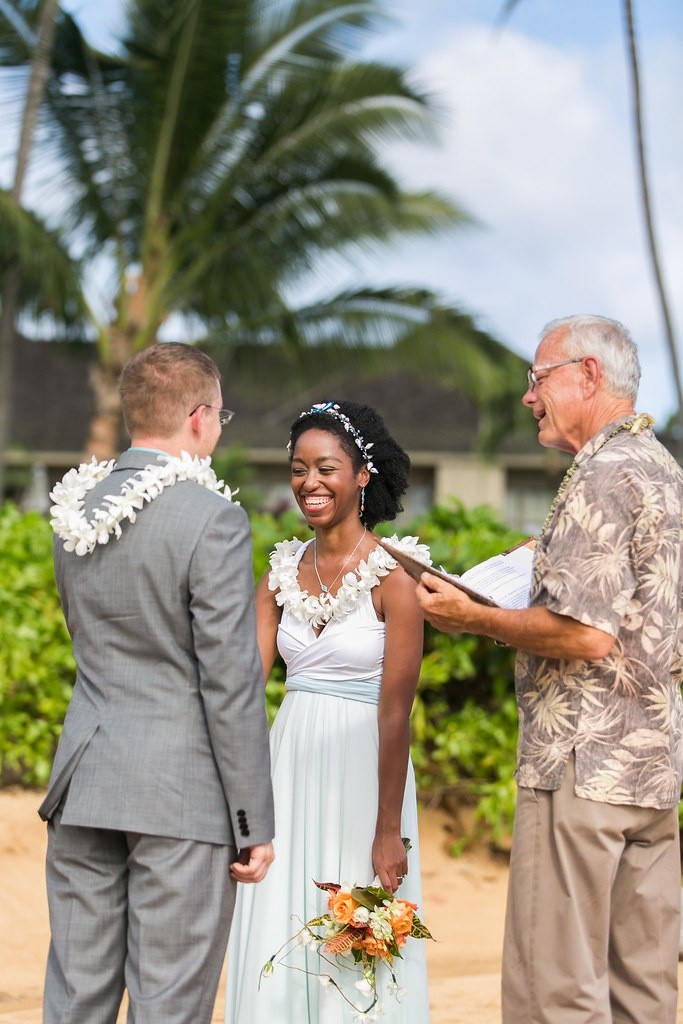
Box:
[{"left": 373, "top": 537, "right": 540, "bottom": 609}]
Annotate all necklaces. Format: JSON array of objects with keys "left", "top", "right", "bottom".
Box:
[
  {"left": 314, "top": 526, "right": 366, "bottom": 592},
  {"left": 539, "top": 419, "right": 636, "bottom": 536}
]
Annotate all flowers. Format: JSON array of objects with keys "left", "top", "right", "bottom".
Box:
[{"left": 258, "top": 839, "right": 435, "bottom": 1024}]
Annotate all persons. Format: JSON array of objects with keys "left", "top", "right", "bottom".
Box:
[
  {"left": 43, "top": 342, "right": 276, "bottom": 1024},
  {"left": 226, "top": 400, "right": 434, "bottom": 1024},
  {"left": 418, "top": 315, "right": 683, "bottom": 1023}
]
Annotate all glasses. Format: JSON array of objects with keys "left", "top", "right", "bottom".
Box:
[
  {"left": 189, "top": 404, "right": 235, "bottom": 426},
  {"left": 527, "top": 358, "right": 603, "bottom": 392}
]
